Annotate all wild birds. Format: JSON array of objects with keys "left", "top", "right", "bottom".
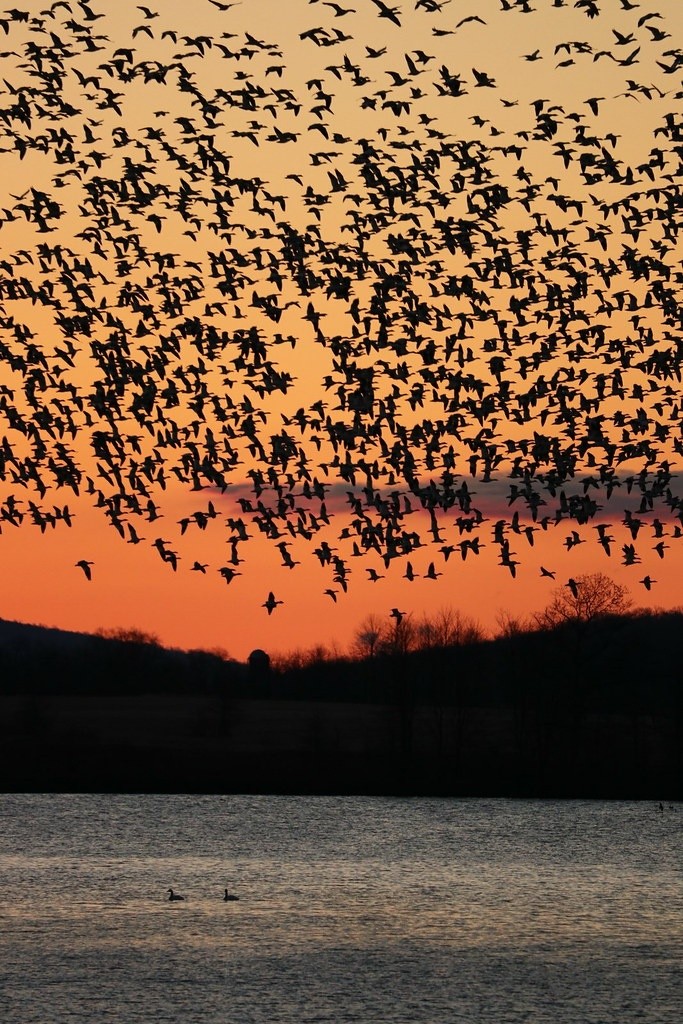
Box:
[{"left": 1, "top": 0, "right": 682, "bottom": 630}]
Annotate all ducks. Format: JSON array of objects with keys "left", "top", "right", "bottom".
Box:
[
  {"left": 223, "top": 889, "right": 240, "bottom": 901},
  {"left": 167, "top": 889, "right": 184, "bottom": 901}
]
